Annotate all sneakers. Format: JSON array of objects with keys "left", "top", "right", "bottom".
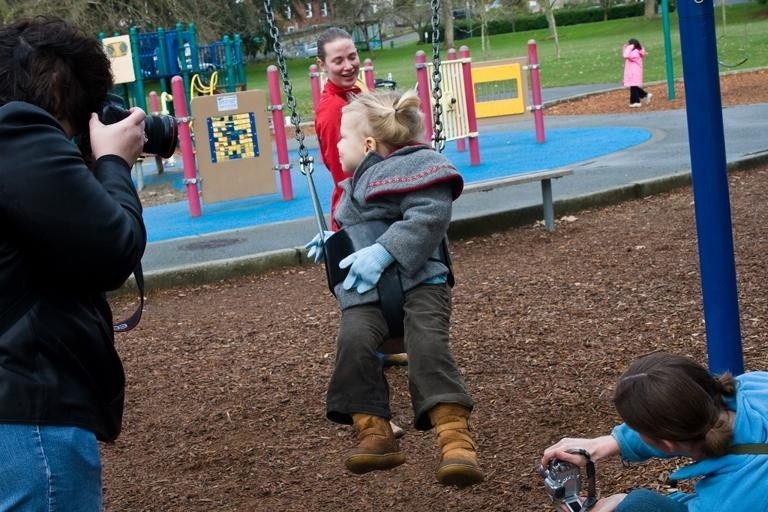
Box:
[
  {"left": 381, "top": 352, "right": 409, "bottom": 367},
  {"left": 628, "top": 102, "right": 642, "bottom": 109},
  {"left": 387, "top": 417, "right": 406, "bottom": 441},
  {"left": 643, "top": 92, "right": 654, "bottom": 106}
]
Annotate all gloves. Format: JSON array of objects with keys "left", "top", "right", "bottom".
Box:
[
  {"left": 303, "top": 230, "right": 336, "bottom": 265},
  {"left": 338, "top": 242, "right": 396, "bottom": 295}
]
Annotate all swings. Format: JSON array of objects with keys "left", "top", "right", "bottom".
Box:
[{"left": 261, "top": 1, "right": 454, "bottom": 355}]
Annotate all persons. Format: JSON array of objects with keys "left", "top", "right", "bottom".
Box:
[
  {"left": 537, "top": 351, "right": 767, "bottom": 512},
  {"left": 302, "top": 86, "right": 487, "bottom": 487},
  {"left": 0, "top": 14, "right": 147, "bottom": 510},
  {"left": 623, "top": 38, "right": 654, "bottom": 109},
  {"left": 313, "top": 27, "right": 410, "bottom": 438}
]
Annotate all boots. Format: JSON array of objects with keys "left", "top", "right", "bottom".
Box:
[
  {"left": 345, "top": 411, "right": 406, "bottom": 474},
  {"left": 428, "top": 402, "right": 484, "bottom": 487}
]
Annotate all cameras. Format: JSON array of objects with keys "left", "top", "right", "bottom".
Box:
[
  {"left": 85, "top": 93, "right": 178, "bottom": 159},
  {"left": 538, "top": 458, "right": 583, "bottom": 512}
]
